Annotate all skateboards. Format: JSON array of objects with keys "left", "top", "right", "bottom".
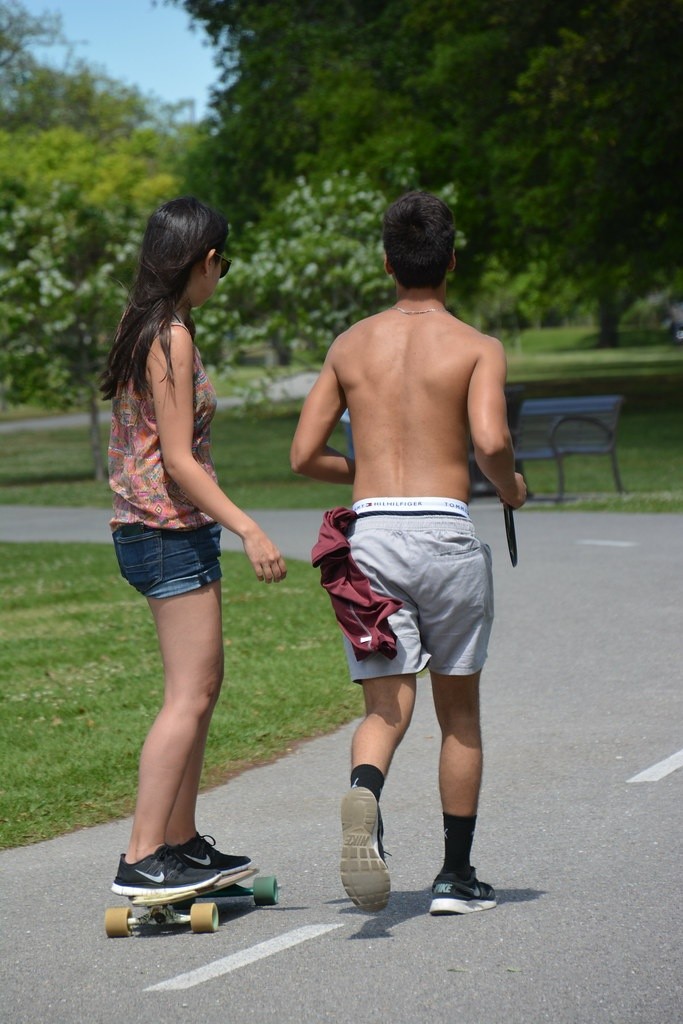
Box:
[{"left": 103, "top": 868, "right": 278, "bottom": 938}]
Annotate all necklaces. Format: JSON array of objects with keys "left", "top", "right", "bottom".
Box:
[{"left": 390, "top": 306, "right": 447, "bottom": 314}]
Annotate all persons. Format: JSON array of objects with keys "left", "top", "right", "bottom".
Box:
[
  {"left": 99, "top": 195, "right": 288, "bottom": 896},
  {"left": 290, "top": 193, "right": 526, "bottom": 915}
]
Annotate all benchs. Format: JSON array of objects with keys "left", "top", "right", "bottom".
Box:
[{"left": 469, "top": 393, "right": 625, "bottom": 504}]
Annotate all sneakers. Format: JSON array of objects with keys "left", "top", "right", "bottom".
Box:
[
  {"left": 341, "top": 778, "right": 392, "bottom": 913},
  {"left": 111, "top": 843, "right": 223, "bottom": 896},
  {"left": 170, "top": 832, "right": 251, "bottom": 876},
  {"left": 429, "top": 867, "right": 497, "bottom": 913}
]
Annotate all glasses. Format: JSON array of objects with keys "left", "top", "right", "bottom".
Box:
[{"left": 214, "top": 252, "right": 232, "bottom": 278}]
